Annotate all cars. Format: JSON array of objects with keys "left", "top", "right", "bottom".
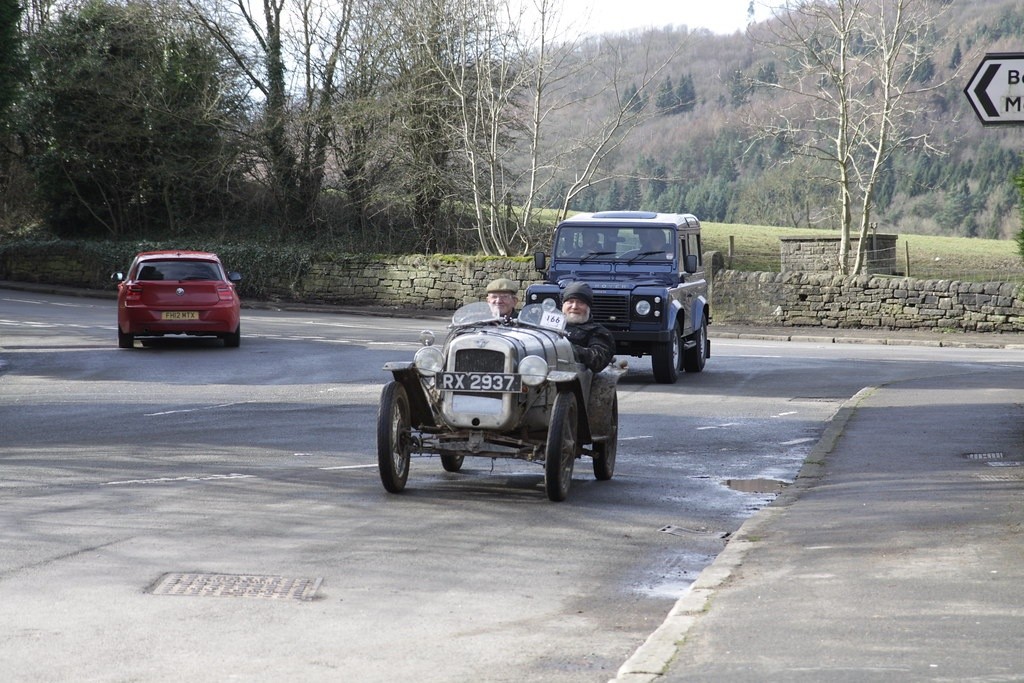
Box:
[{"left": 109, "top": 249, "right": 242, "bottom": 347}]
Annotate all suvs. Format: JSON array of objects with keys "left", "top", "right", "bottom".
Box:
[{"left": 525, "top": 210, "right": 711, "bottom": 384}]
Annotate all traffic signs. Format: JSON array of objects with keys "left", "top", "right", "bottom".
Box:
[{"left": 966, "top": 57, "right": 1023, "bottom": 124}]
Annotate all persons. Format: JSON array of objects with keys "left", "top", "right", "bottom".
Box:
[
  {"left": 640, "top": 229, "right": 666, "bottom": 260},
  {"left": 562, "top": 282, "right": 615, "bottom": 374},
  {"left": 561, "top": 230, "right": 602, "bottom": 258},
  {"left": 485, "top": 278, "right": 519, "bottom": 318}
]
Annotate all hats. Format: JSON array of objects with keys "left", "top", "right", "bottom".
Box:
[
  {"left": 486, "top": 278, "right": 518, "bottom": 294},
  {"left": 562, "top": 282, "right": 593, "bottom": 307}
]
